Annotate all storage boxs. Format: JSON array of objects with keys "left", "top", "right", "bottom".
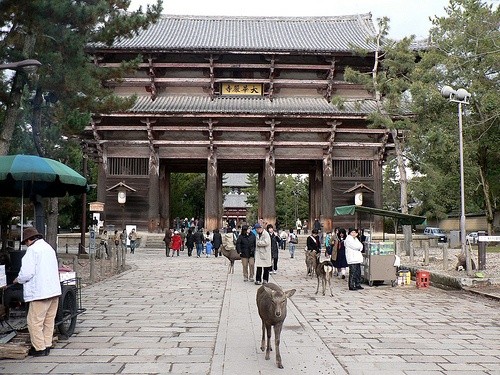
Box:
[{"left": 60, "top": 271, "right": 76, "bottom": 286}]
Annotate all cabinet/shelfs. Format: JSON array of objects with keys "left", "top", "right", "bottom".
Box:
[{"left": 362, "top": 242, "right": 397, "bottom": 281}]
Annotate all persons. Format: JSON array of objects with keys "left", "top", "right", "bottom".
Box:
[
  {"left": 14, "top": 227, "right": 62, "bottom": 356},
  {"left": 307, "top": 229, "right": 321, "bottom": 276},
  {"left": 344, "top": 229, "right": 364, "bottom": 291},
  {"left": 296, "top": 217, "right": 302, "bottom": 235},
  {"left": 186, "top": 229, "right": 222, "bottom": 258},
  {"left": 101, "top": 230, "right": 109, "bottom": 240},
  {"left": 267, "top": 224, "right": 283, "bottom": 274},
  {"left": 225, "top": 228, "right": 237, "bottom": 274},
  {"left": 120, "top": 229, "right": 127, "bottom": 254},
  {"left": 173, "top": 217, "right": 204, "bottom": 233},
  {"left": 252, "top": 223, "right": 272, "bottom": 285},
  {"left": 113, "top": 230, "right": 121, "bottom": 242},
  {"left": 129, "top": 228, "right": 138, "bottom": 254},
  {"left": 288, "top": 228, "right": 297, "bottom": 258},
  {"left": 312, "top": 218, "right": 320, "bottom": 230},
  {"left": 280, "top": 229, "right": 288, "bottom": 250},
  {"left": 163, "top": 228, "right": 185, "bottom": 257},
  {"left": 325, "top": 226, "right": 366, "bottom": 279},
  {"left": 236, "top": 225, "right": 256, "bottom": 282}
]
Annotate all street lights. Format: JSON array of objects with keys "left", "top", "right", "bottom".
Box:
[{"left": 440, "top": 85, "right": 472, "bottom": 255}]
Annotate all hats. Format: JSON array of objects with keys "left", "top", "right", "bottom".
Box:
[
  {"left": 349, "top": 228, "right": 356, "bottom": 234},
  {"left": 20, "top": 228, "right": 44, "bottom": 244},
  {"left": 252, "top": 223, "right": 262, "bottom": 229}
]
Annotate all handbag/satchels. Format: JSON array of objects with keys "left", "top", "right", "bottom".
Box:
[{"left": 291, "top": 238, "right": 298, "bottom": 244}]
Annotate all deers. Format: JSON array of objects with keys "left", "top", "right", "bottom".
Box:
[
  {"left": 314, "top": 252, "right": 335, "bottom": 297},
  {"left": 304, "top": 250, "right": 317, "bottom": 281},
  {"left": 256, "top": 282, "right": 297, "bottom": 369},
  {"left": 217, "top": 244, "right": 241, "bottom": 274}
]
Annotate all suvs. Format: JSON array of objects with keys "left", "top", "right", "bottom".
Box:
[{"left": 423, "top": 226, "right": 448, "bottom": 243}]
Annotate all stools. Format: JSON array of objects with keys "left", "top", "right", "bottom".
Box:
[
  {"left": 399, "top": 271, "right": 411, "bottom": 284},
  {"left": 415, "top": 270, "right": 429, "bottom": 288}
]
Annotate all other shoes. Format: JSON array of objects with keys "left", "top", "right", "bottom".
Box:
[
  {"left": 28, "top": 346, "right": 45, "bottom": 356},
  {"left": 269, "top": 271, "right": 272, "bottom": 274},
  {"left": 255, "top": 281, "right": 261, "bottom": 284},
  {"left": 356, "top": 285, "right": 364, "bottom": 289},
  {"left": 263, "top": 280, "right": 268, "bottom": 284},
  {"left": 349, "top": 287, "right": 357, "bottom": 290},
  {"left": 249, "top": 277, "right": 254, "bottom": 282},
  {"left": 274, "top": 270, "right": 276, "bottom": 274},
  {"left": 44, "top": 347, "right": 50, "bottom": 355},
  {"left": 244, "top": 278, "right": 248, "bottom": 281}
]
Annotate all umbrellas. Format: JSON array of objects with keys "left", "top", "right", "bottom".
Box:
[{"left": 0, "top": 155, "right": 93, "bottom": 242}]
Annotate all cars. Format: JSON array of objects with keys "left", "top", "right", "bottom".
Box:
[{"left": 466, "top": 232, "right": 479, "bottom": 245}]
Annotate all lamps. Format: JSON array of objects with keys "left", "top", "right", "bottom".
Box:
[
  {"left": 118, "top": 190, "right": 126, "bottom": 203},
  {"left": 355, "top": 192, "right": 363, "bottom": 206}
]
